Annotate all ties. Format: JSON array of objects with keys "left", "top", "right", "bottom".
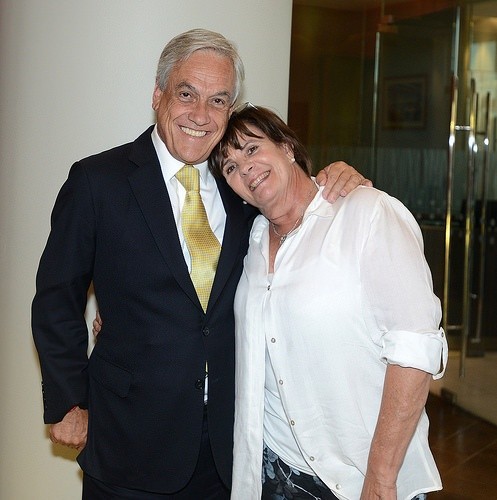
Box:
[{"left": 174, "top": 164, "right": 223, "bottom": 315}]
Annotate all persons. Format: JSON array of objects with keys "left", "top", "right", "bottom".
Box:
[
  {"left": 32, "top": 29, "right": 372, "bottom": 500},
  {"left": 92, "top": 107, "right": 447, "bottom": 500}
]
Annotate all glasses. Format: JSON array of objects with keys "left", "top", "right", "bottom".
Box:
[{"left": 232, "top": 101, "right": 260, "bottom": 115}]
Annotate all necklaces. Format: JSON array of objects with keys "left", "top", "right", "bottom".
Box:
[{"left": 272, "top": 185, "right": 315, "bottom": 243}]
